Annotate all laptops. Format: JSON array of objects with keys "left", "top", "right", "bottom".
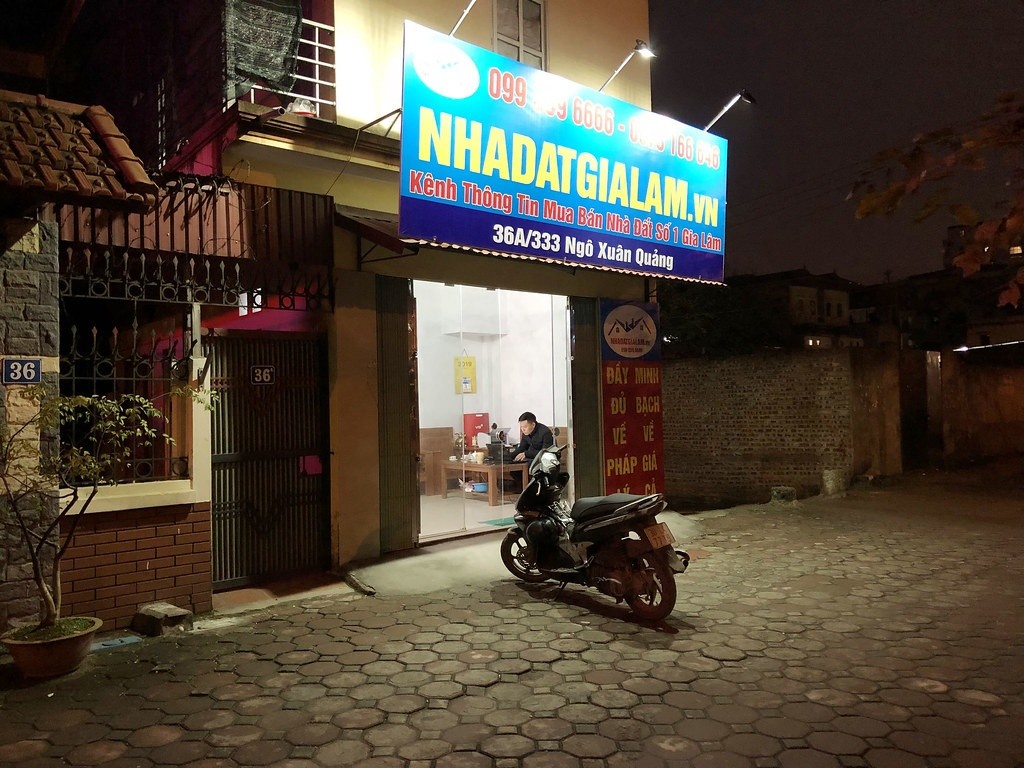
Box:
[{"left": 486, "top": 444, "right": 528, "bottom": 464}]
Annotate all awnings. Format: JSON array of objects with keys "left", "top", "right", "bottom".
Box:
[{"left": 335, "top": 203, "right": 728, "bottom": 288}]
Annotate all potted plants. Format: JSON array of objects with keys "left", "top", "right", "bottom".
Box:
[{"left": 0, "top": 385, "right": 222, "bottom": 679}]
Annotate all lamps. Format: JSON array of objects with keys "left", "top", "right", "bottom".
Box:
[
  {"left": 703, "top": 86, "right": 755, "bottom": 132},
  {"left": 597, "top": 38, "right": 657, "bottom": 94}
]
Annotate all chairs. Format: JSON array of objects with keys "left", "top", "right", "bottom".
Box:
[
  {"left": 420, "top": 427, "right": 472, "bottom": 495},
  {"left": 520, "top": 426, "right": 569, "bottom": 495}
]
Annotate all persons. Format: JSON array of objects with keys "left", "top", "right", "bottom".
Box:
[{"left": 505, "top": 412, "right": 562, "bottom": 494}]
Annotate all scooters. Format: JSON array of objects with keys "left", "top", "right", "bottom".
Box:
[{"left": 500, "top": 428, "right": 689, "bottom": 623}]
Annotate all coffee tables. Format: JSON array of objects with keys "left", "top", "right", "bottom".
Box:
[{"left": 440, "top": 459, "right": 529, "bottom": 506}]
